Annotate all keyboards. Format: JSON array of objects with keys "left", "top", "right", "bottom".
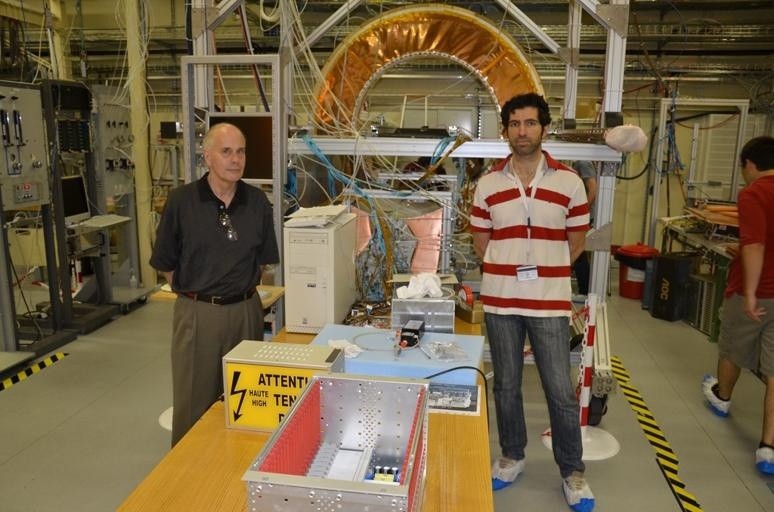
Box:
[{"left": 221, "top": 334, "right": 344, "bottom": 370}]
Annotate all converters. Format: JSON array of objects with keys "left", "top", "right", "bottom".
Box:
[{"left": 401, "top": 320, "right": 426, "bottom": 347}]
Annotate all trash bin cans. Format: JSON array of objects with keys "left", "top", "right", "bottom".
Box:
[{"left": 613, "top": 240, "right": 659, "bottom": 298}]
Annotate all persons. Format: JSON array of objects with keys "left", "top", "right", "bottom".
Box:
[
  {"left": 703, "top": 135, "right": 774, "bottom": 473},
  {"left": 149, "top": 122, "right": 279, "bottom": 448},
  {"left": 571, "top": 162, "right": 599, "bottom": 295},
  {"left": 470, "top": 91, "right": 597, "bottom": 512}
]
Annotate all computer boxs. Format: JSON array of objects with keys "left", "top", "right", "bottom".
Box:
[{"left": 282, "top": 214, "right": 359, "bottom": 334}]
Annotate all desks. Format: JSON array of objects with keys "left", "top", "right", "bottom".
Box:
[
  {"left": 112, "top": 301, "right": 494, "bottom": 512},
  {"left": 151, "top": 283, "right": 285, "bottom": 310},
  {"left": 656, "top": 214, "right": 740, "bottom": 335}
]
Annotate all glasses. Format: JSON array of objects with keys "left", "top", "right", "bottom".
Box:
[{"left": 218, "top": 207, "right": 239, "bottom": 244}]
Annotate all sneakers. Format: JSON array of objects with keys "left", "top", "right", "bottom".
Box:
[
  {"left": 560, "top": 469, "right": 597, "bottom": 512},
  {"left": 754, "top": 444, "right": 774, "bottom": 475},
  {"left": 490, "top": 455, "right": 527, "bottom": 492},
  {"left": 699, "top": 372, "right": 731, "bottom": 418}
]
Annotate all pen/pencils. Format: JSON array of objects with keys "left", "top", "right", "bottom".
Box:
[{"left": 419, "top": 346, "right": 432, "bottom": 359}]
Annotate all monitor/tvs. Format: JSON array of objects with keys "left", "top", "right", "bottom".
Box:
[{"left": 198, "top": 110, "right": 277, "bottom": 187}]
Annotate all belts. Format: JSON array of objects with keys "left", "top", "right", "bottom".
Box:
[{"left": 178, "top": 284, "right": 257, "bottom": 307}]
